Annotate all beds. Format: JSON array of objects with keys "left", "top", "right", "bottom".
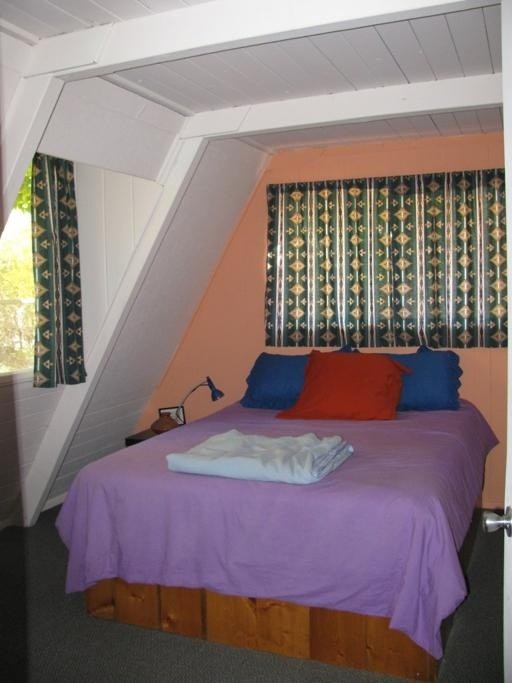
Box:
[{"left": 74, "top": 396, "right": 492, "bottom": 681}]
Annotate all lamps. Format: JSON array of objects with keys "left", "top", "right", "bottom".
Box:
[{"left": 174, "top": 376, "right": 226, "bottom": 417}]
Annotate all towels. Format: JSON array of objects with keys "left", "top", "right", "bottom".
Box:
[{"left": 165, "top": 427, "right": 355, "bottom": 486}]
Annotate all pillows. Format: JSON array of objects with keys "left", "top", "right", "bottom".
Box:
[{"left": 241, "top": 345, "right": 463, "bottom": 421}]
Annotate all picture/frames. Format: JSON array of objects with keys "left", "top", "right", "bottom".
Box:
[{"left": 158, "top": 405, "right": 186, "bottom": 426}]
